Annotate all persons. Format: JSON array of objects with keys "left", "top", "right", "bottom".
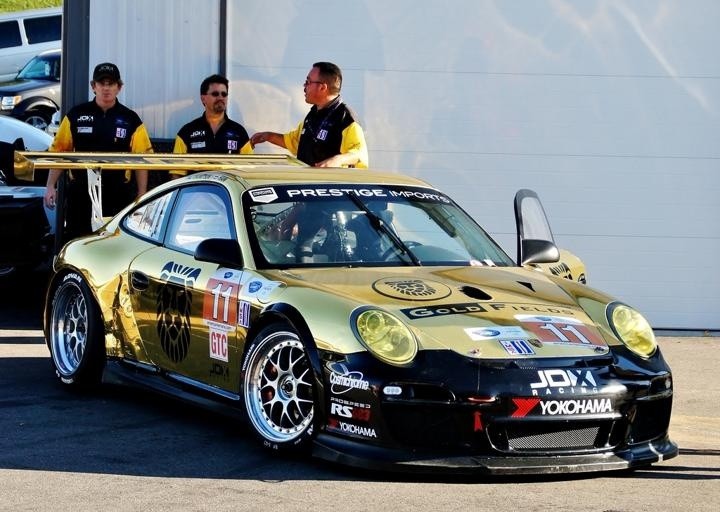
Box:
[
  {"left": 250, "top": 62, "right": 369, "bottom": 168},
  {"left": 169, "top": 74, "right": 253, "bottom": 178},
  {"left": 43, "top": 62, "right": 155, "bottom": 238}
]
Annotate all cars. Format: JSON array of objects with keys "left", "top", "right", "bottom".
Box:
[{"left": 0, "top": 49, "right": 61, "bottom": 136}]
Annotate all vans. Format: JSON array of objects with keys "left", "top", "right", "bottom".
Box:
[{"left": 0, "top": 6, "right": 63, "bottom": 82}]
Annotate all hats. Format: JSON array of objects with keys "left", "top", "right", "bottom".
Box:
[{"left": 93, "top": 62, "right": 122, "bottom": 82}]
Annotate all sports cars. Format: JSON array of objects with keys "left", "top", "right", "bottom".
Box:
[{"left": 13, "top": 153, "right": 678, "bottom": 476}]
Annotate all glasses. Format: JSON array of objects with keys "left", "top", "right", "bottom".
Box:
[
  {"left": 306, "top": 79, "right": 324, "bottom": 86},
  {"left": 212, "top": 91, "right": 228, "bottom": 98}
]
